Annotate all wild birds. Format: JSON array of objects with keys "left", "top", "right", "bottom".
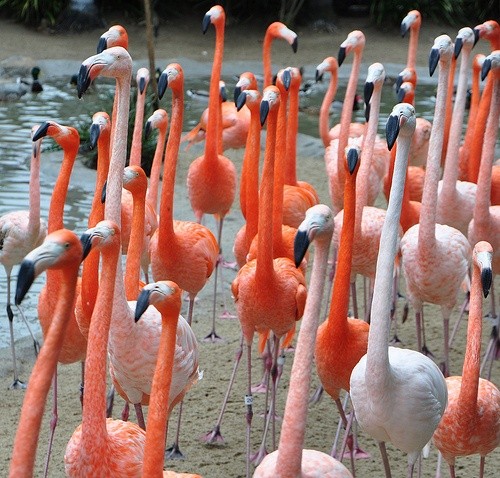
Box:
[{"left": 1, "top": 0, "right": 499, "bottom": 478}]
